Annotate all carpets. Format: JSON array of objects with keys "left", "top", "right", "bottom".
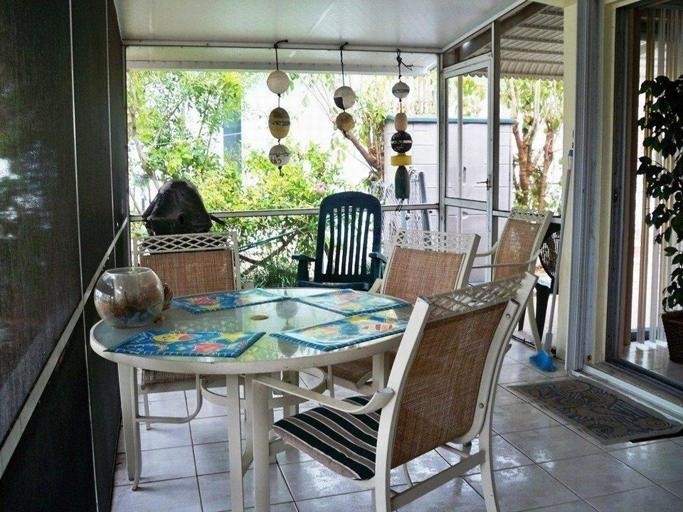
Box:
[{"left": 505, "top": 375, "right": 683, "bottom": 445}]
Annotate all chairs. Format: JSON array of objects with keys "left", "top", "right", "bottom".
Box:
[
  {"left": 292, "top": 191, "right": 387, "bottom": 302},
  {"left": 129, "top": 228, "right": 246, "bottom": 490},
  {"left": 467, "top": 206, "right": 553, "bottom": 355},
  {"left": 248, "top": 274, "right": 538, "bottom": 512},
  {"left": 290, "top": 227, "right": 482, "bottom": 449}
]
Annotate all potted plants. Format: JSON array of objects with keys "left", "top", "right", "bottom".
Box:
[{"left": 637, "top": 71, "right": 683, "bottom": 363}]
